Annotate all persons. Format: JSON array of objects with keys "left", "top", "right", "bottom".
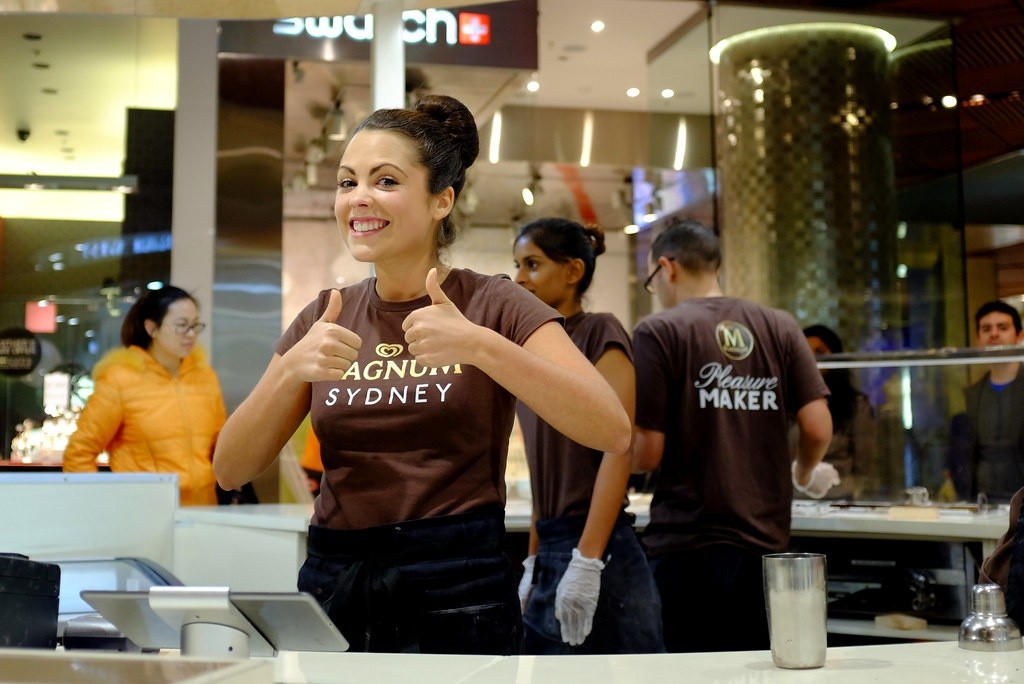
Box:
[
  {"left": 961, "top": 295, "right": 1024, "bottom": 512},
  {"left": 208, "top": 89, "right": 633, "bottom": 656},
  {"left": 61, "top": 282, "right": 231, "bottom": 507},
  {"left": 787, "top": 321, "right": 889, "bottom": 505},
  {"left": 616, "top": 218, "right": 842, "bottom": 652},
  {"left": 505, "top": 215, "right": 666, "bottom": 654}
]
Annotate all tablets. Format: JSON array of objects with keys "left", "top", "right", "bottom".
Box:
[{"left": 80, "top": 588, "right": 350, "bottom": 653}]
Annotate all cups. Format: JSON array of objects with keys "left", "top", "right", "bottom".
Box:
[{"left": 763, "top": 552, "right": 829, "bottom": 670}]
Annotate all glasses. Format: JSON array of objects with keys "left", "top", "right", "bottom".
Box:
[
  {"left": 644, "top": 255, "right": 676, "bottom": 295},
  {"left": 162, "top": 321, "right": 206, "bottom": 335}
]
